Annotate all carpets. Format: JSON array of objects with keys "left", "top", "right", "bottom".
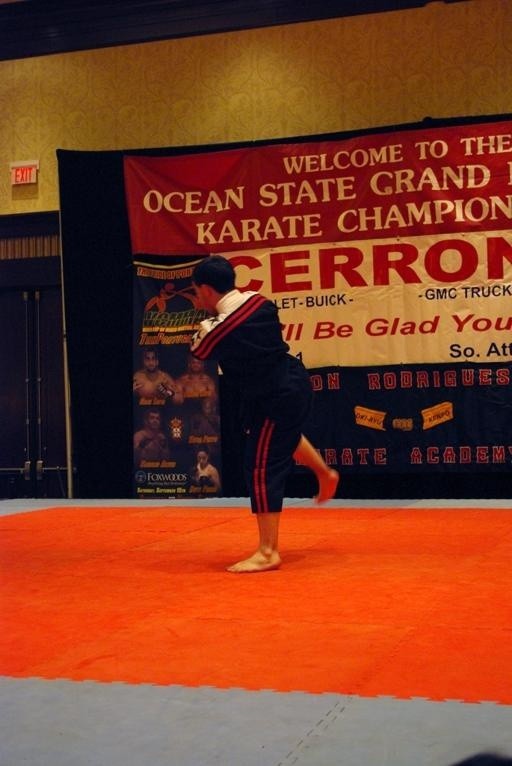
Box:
[{"left": 1, "top": 505, "right": 512, "bottom": 708}]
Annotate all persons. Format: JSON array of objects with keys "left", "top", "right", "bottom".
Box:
[
  {"left": 190, "top": 255, "right": 342, "bottom": 572},
  {"left": 134, "top": 408, "right": 171, "bottom": 467},
  {"left": 188, "top": 398, "right": 221, "bottom": 440},
  {"left": 133, "top": 347, "right": 184, "bottom": 406},
  {"left": 188, "top": 447, "right": 220, "bottom": 493},
  {"left": 172, "top": 354, "right": 220, "bottom": 407}
]
